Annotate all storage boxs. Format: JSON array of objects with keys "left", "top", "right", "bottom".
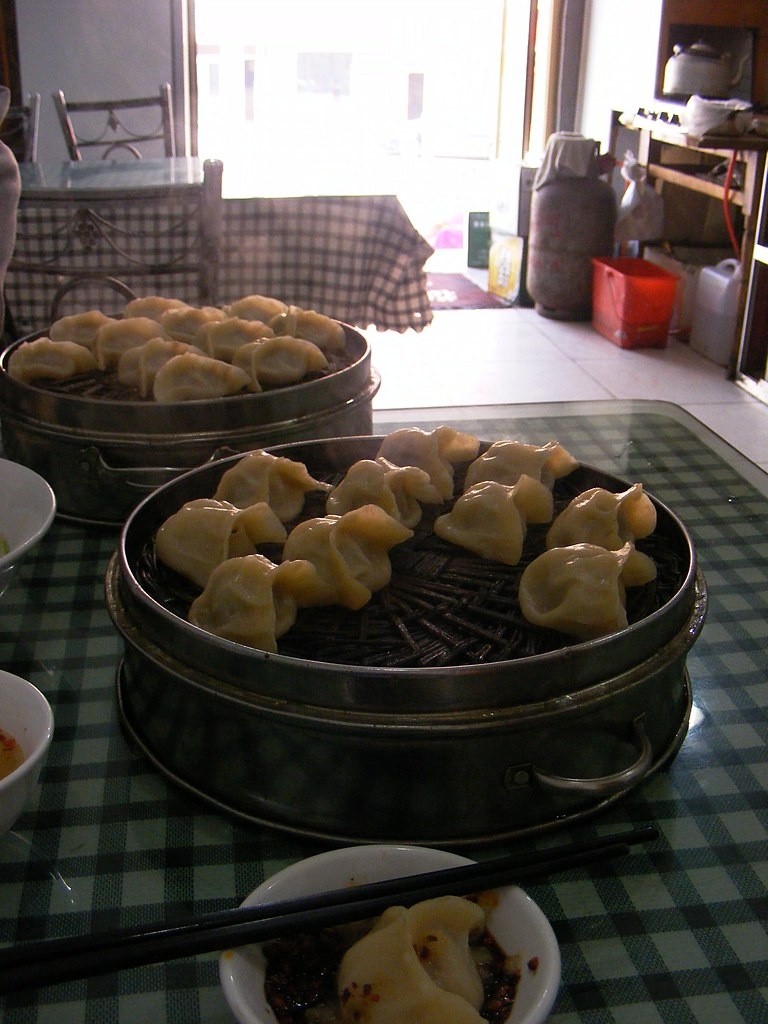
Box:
[
  {"left": 467, "top": 211, "right": 493, "bottom": 268},
  {"left": 488, "top": 232, "right": 534, "bottom": 307},
  {"left": 591, "top": 256, "right": 682, "bottom": 349}
]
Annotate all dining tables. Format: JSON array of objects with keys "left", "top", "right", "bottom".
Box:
[
  {"left": 2, "top": 148, "right": 435, "bottom": 336},
  {"left": 0, "top": 398, "right": 768, "bottom": 1024}
]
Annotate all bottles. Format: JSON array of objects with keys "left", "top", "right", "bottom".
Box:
[{"left": 689, "top": 258, "right": 742, "bottom": 365}]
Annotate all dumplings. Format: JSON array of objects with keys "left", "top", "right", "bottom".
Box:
[
  {"left": 155, "top": 424, "right": 657, "bottom": 655},
  {"left": 336, "top": 895, "right": 490, "bottom": 1024},
  {"left": 6, "top": 295, "right": 348, "bottom": 403}
]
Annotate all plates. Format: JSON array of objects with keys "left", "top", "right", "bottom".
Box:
[{"left": 222, "top": 846, "right": 559, "bottom": 1024}]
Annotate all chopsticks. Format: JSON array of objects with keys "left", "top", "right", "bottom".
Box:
[{"left": 0, "top": 825, "right": 661, "bottom": 992}]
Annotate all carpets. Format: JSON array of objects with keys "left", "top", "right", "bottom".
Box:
[{"left": 421, "top": 271, "right": 514, "bottom": 309}]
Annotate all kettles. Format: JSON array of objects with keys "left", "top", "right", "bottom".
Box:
[{"left": 663, "top": 38, "right": 751, "bottom": 99}]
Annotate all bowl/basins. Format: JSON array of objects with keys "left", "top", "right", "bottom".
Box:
[
  {"left": 0, "top": 669, "right": 54, "bottom": 839},
  {"left": 0, "top": 458, "right": 56, "bottom": 597}
]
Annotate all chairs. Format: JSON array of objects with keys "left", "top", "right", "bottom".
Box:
[
  {"left": 0, "top": 88, "right": 42, "bottom": 162},
  {"left": 2, "top": 159, "right": 223, "bottom": 341},
  {"left": 51, "top": 80, "right": 174, "bottom": 163}
]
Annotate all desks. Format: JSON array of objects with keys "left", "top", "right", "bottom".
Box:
[{"left": 632, "top": 115, "right": 767, "bottom": 383}]
